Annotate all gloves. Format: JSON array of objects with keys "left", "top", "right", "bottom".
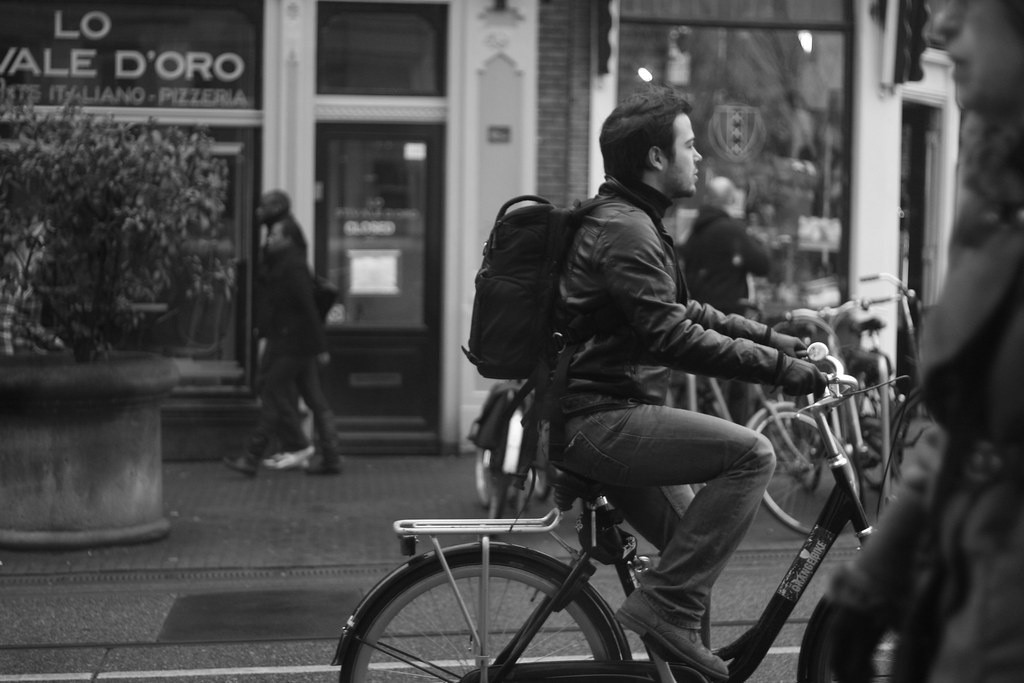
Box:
[
  {"left": 771, "top": 328, "right": 807, "bottom": 360},
  {"left": 775, "top": 353, "right": 826, "bottom": 404}
]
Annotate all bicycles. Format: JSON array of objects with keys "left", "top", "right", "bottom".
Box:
[
  {"left": 331, "top": 343, "right": 881, "bottom": 683},
  {"left": 471, "top": 273, "right": 958, "bottom": 535}
]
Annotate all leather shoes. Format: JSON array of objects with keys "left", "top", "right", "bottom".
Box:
[{"left": 615, "top": 583, "right": 738, "bottom": 678}]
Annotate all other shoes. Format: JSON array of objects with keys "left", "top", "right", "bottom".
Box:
[
  {"left": 219, "top": 448, "right": 262, "bottom": 479},
  {"left": 263, "top": 444, "right": 313, "bottom": 473},
  {"left": 302, "top": 451, "right": 348, "bottom": 477}
]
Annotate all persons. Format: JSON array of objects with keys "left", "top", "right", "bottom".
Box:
[
  {"left": 221, "top": 191, "right": 346, "bottom": 477},
  {"left": 915, "top": 0, "right": 1024, "bottom": 683},
  {"left": 539, "top": 87, "right": 830, "bottom": 683},
  {"left": 682, "top": 176, "right": 770, "bottom": 427}
]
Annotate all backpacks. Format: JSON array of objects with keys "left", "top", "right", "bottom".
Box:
[{"left": 460, "top": 193, "right": 692, "bottom": 504}]
